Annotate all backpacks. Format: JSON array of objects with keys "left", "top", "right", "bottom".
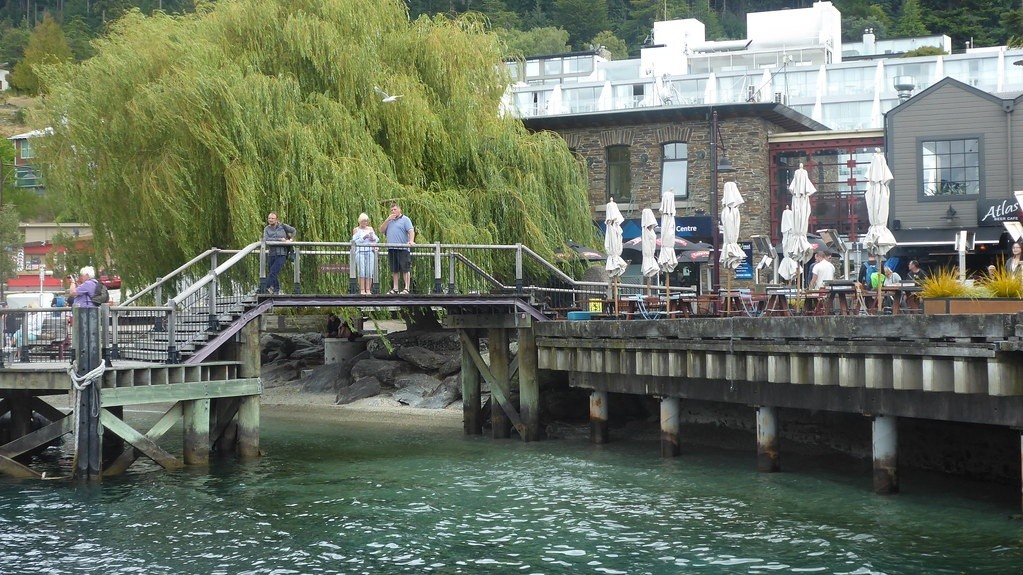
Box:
[
  {"left": 84, "top": 279, "right": 109, "bottom": 307},
  {"left": 863, "top": 261, "right": 879, "bottom": 287}
]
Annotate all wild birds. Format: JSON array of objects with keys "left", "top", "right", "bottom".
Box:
[{"left": 374, "top": 87, "right": 403, "bottom": 103}]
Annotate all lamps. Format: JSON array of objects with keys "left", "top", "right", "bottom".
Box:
[{"left": 940, "top": 203, "right": 960, "bottom": 223}]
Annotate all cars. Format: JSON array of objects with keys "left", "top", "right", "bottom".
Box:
[
  {"left": 98, "top": 269, "right": 121, "bottom": 290},
  {"left": 105, "top": 287, "right": 136, "bottom": 307}
]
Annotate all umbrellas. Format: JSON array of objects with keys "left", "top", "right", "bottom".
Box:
[
  {"left": 657, "top": 190, "right": 677, "bottom": 315},
  {"left": 863, "top": 146, "right": 896, "bottom": 312},
  {"left": 605, "top": 197, "right": 626, "bottom": 319},
  {"left": 788, "top": 162, "right": 814, "bottom": 313},
  {"left": 782, "top": 205, "right": 803, "bottom": 283},
  {"left": 721, "top": 181, "right": 747, "bottom": 317},
  {"left": 640, "top": 208, "right": 660, "bottom": 297},
  {"left": 549, "top": 234, "right": 715, "bottom": 281},
  {"left": 774, "top": 232, "right": 842, "bottom": 257}
]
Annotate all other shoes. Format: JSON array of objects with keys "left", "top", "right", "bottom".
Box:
[
  {"left": 360, "top": 290, "right": 366, "bottom": 294},
  {"left": 274, "top": 290, "right": 280, "bottom": 295},
  {"left": 366, "top": 289, "right": 372, "bottom": 294}
]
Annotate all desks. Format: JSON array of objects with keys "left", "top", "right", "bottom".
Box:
[{"left": 609, "top": 279, "right": 925, "bottom": 321}]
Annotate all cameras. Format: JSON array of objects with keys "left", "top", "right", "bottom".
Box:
[{"left": 67, "top": 275, "right": 75, "bottom": 279}]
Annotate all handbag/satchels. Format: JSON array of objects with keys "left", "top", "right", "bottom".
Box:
[{"left": 282, "top": 224, "right": 295, "bottom": 262}]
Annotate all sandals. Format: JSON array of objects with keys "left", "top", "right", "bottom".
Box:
[
  {"left": 386, "top": 289, "right": 399, "bottom": 294},
  {"left": 400, "top": 288, "right": 410, "bottom": 294}
]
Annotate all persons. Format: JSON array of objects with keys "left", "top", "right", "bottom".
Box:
[
  {"left": 1005, "top": 242, "right": 1022, "bottom": 279},
  {"left": 353, "top": 212, "right": 379, "bottom": 294},
  {"left": 8, "top": 266, "right": 96, "bottom": 346},
  {"left": 378, "top": 203, "right": 414, "bottom": 294},
  {"left": 809, "top": 251, "right": 835, "bottom": 290},
  {"left": 264, "top": 213, "right": 295, "bottom": 294},
  {"left": 858, "top": 252, "right": 927, "bottom": 305}
]
